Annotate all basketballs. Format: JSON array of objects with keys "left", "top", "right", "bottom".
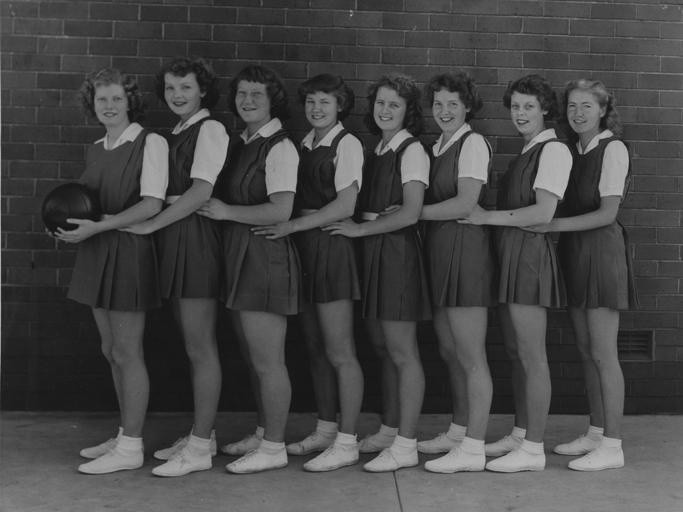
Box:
[{"left": 41, "top": 183, "right": 101, "bottom": 239}]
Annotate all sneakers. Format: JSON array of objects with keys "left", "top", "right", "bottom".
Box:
[
  {"left": 363, "top": 446, "right": 420, "bottom": 474},
  {"left": 418, "top": 433, "right": 462, "bottom": 453},
  {"left": 79, "top": 448, "right": 147, "bottom": 475},
  {"left": 424, "top": 447, "right": 487, "bottom": 475},
  {"left": 486, "top": 436, "right": 519, "bottom": 459},
  {"left": 553, "top": 436, "right": 597, "bottom": 456},
  {"left": 486, "top": 449, "right": 548, "bottom": 474},
  {"left": 222, "top": 433, "right": 263, "bottom": 456},
  {"left": 302, "top": 444, "right": 360, "bottom": 472},
  {"left": 225, "top": 449, "right": 289, "bottom": 475},
  {"left": 153, "top": 454, "right": 213, "bottom": 477},
  {"left": 155, "top": 431, "right": 220, "bottom": 462},
  {"left": 568, "top": 449, "right": 625, "bottom": 473},
  {"left": 286, "top": 431, "right": 334, "bottom": 456},
  {"left": 77, "top": 439, "right": 120, "bottom": 459},
  {"left": 358, "top": 432, "right": 394, "bottom": 453}
]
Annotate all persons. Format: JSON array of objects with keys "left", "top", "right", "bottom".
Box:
[
  {"left": 117, "top": 57, "right": 230, "bottom": 477},
  {"left": 41, "top": 67, "right": 169, "bottom": 475},
  {"left": 376, "top": 72, "right": 495, "bottom": 474},
  {"left": 457, "top": 73, "right": 572, "bottom": 474},
  {"left": 321, "top": 68, "right": 431, "bottom": 473},
  {"left": 250, "top": 73, "right": 364, "bottom": 473},
  {"left": 198, "top": 65, "right": 301, "bottom": 475},
  {"left": 522, "top": 78, "right": 638, "bottom": 473}
]
